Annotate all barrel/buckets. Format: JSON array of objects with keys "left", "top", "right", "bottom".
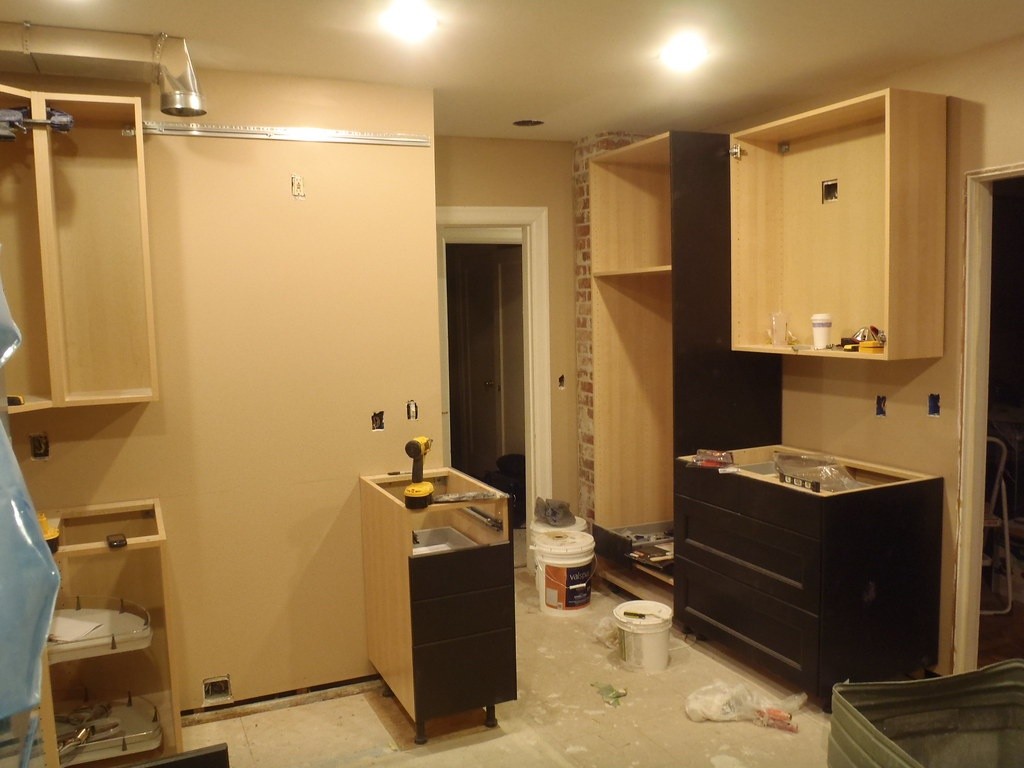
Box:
[
  {"left": 533, "top": 531, "right": 596, "bottom": 615},
  {"left": 530, "top": 516, "right": 588, "bottom": 591},
  {"left": 613, "top": 600, "right": 673, "bottom": 672}
]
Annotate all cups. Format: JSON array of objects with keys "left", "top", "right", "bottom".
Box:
[
  {"left": 772, "top": 313, "right": 785, "bottom": 345},
  {"left": 812, "top": 314, "right": 832, "bottom": 349}
]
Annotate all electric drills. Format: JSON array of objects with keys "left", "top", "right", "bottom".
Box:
[{"left": 402, "top": 436, "right": 436, "bottom": 511}]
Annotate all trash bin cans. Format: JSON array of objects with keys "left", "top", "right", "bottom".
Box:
[{"left": 490, "top": 454, "right": 526, "bottom": 528}]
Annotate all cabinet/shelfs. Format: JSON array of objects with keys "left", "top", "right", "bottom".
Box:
[
  {"left": 0, "top": 84, "right": 160, "bottom": 418},
  {"left": 587, "top": 129, "right": 782, "bottom": 634},
  {"left": 357, "top": 466, "right": 519, "bottom": 745},
  {"left": 40, "top": 497, "right": 230, "bottom": 768},
  {"left": 674, "top": 444, "right": 946, "bottom": 715}
]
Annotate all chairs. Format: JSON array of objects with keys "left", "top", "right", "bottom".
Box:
[{"left": 979, "top": 435, "right": 1013, "bottom": 617}]
